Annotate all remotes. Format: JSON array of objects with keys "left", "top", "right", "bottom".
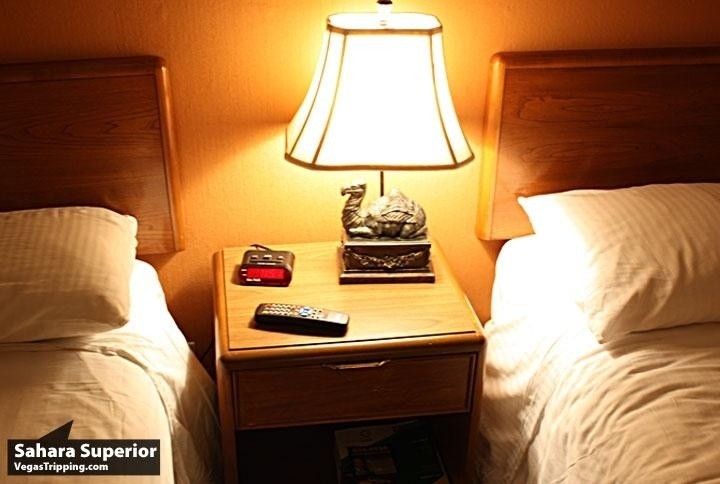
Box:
[{"left": 255, "top": 303, "right": 349, "bottom": 329}]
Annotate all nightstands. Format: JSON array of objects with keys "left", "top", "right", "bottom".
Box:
[{"left": 209, "top": 240, "right": 487, "bottom": 484}]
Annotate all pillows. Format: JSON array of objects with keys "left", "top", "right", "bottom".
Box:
[
  {"left": 516, "top": 183, "right": 718, "bottom": 346},
  {"left": 0, "top": 204, "right": 138, "bottom": 343}
]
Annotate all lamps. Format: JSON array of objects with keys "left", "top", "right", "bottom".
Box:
[{"left": 280, "top": 11, "right": 480, "bottom": 285}]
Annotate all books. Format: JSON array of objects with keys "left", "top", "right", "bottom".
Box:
[{"left": 334, "top": 420, "right": 450, "bottom": 484}]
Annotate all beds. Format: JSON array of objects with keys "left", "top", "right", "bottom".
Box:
[
  {"left": 491, "top": 47, "right": 720, "bottom": 484},
  {"left": 0, "top": 58, "right": 199, "bottom": 484}
]
